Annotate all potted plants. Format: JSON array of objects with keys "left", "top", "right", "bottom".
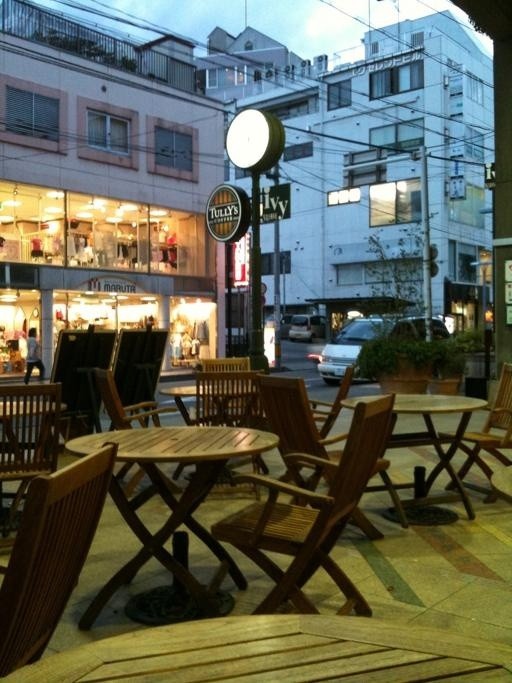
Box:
[
  {"left": 356, "top": 338, "right": 441, "bottom": 395},
  {"left": 432, "top": 327, "right": 487, "bottom": 394}
]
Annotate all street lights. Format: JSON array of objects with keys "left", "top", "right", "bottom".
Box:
[
  {"left": 225, "top": 107, "right": 286, "bottom": 375},
  {"left": 471, "top": 260, "right": 493, "bottom": 331}
]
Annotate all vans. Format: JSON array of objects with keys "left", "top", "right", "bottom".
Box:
[
  {"left": 289, "top": 314, "right": 327, "bottom": 340},
  {"left": 316, "top": 316, "right": 452, "bottom": 385},
  {"left": 261, "top": 312, "right": 292, "bottom": 338}
]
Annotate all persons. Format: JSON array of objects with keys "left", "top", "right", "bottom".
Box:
[
  {"left": 171, "top": 329, "right": 180, "bottom": 358},
  {"left": 192, "top": 336, "right": 200, "bottom": 359},
  {"left": 31, "top": 233, "right": 43, "bottom": 261},
  {"left": 75, "top": 233, "right": 88, "bottom": 263},
  {"left": 181, "top": 331, "right": 192, "bottom": 355},
  {"left": 24, "top": 327, "right": 46, "bottom": 384}
]
